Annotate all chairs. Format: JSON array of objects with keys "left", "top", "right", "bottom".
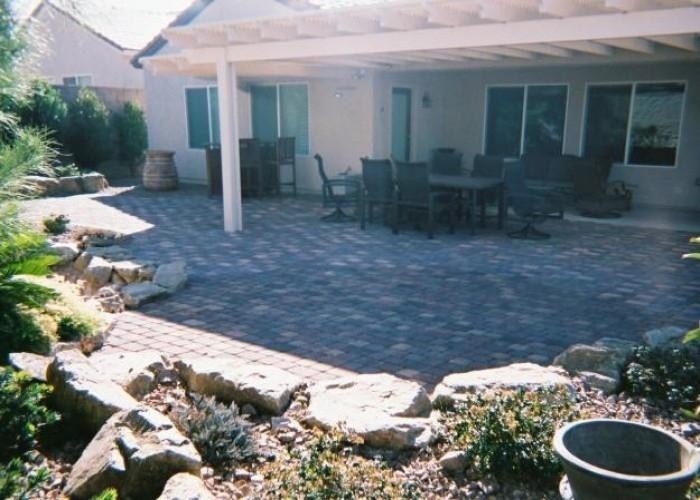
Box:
[{"left": 203, "top": 135, "right": 297, "bottom": 201}]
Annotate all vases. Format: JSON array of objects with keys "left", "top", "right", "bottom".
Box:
[{"left": 552, "top": 418, "right": 700, "bottom": 500}]
